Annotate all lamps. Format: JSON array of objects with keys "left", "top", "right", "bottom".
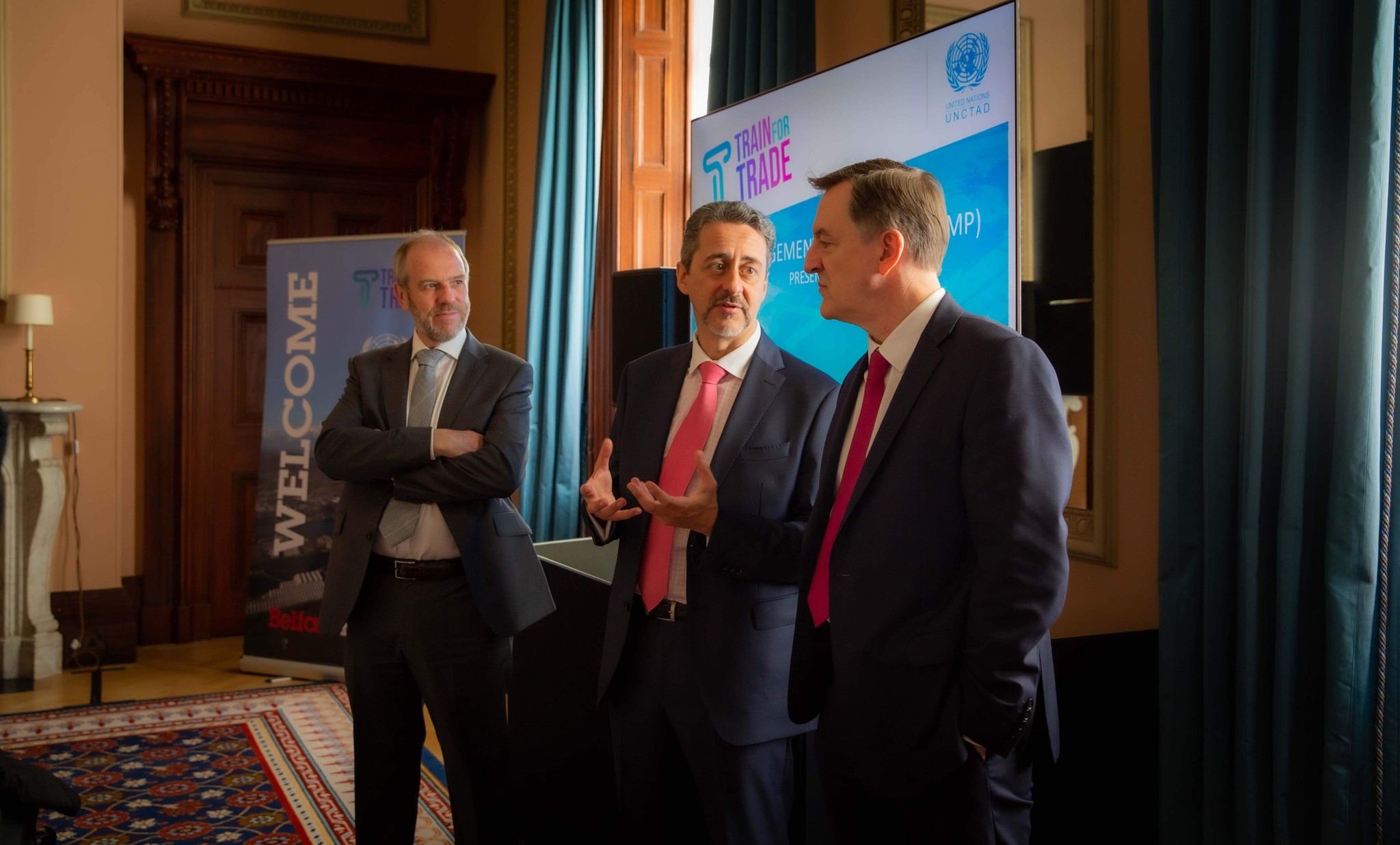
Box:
[{"left": 4, "top": 293, "right": 66, "bottom": 403}]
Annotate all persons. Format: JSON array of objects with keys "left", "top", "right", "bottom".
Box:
[
  {"left": 787, "top": 158, "right": 1073, "bottom": 845},
  {"left": 580, "top": 202, "right": 842, "bottom": 845},
  {"left": 315, "top": 229, "right": 556, "bottom": 845}
]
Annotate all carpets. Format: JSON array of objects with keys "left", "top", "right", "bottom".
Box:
[{"left": 0, "top": 681, "right": 458, "bottom": 845}]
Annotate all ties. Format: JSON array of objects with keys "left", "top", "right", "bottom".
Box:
[
  {"left": 636, "top": 361, "right": 730, "bottom": 612},
  {"left": 379, "top": 350, "right": 446, "bottom": 549},
  {"left": 807, "top": 350, "right": 892, "bottom": 628}
]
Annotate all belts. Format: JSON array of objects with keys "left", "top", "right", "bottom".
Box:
[
  {"left": 366, "top": 552, "right": 466, "bottom": 579},
  {"left": 630, "top": 596, "right": 687, "bottom": 622}
]
